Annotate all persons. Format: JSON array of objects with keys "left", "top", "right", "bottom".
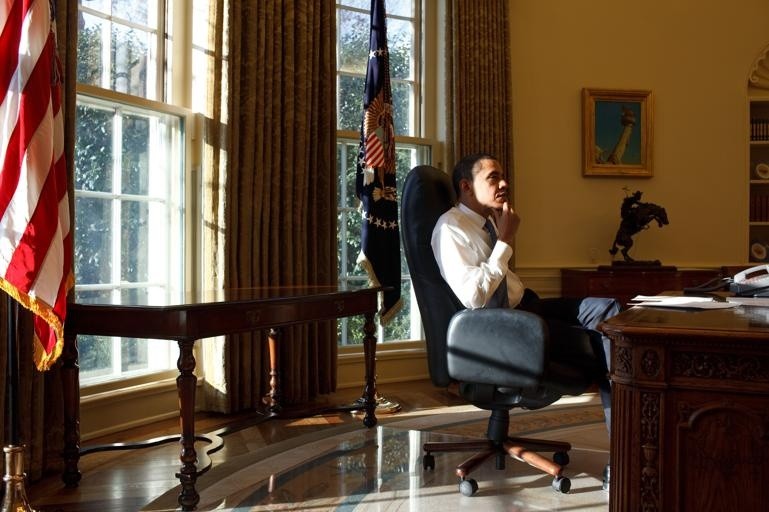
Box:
[{"left": 429, "top": 154, "right": 623, "bottom": 493}]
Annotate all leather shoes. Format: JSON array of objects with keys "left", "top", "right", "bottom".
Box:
[{"left": 603, "top": 464, "right": 610, "bottom": 491}]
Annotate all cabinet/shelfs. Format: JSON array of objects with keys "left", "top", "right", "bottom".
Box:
[
  {"left": 560, "top": 265, "right": 769, "bottom": 311},
  {"left": 747, "top": 96, "right": 769, "bottom": 264}
]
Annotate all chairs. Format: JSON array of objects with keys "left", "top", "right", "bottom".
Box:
[{"left": 400, "top": 165, "right": 592, "bottom": 496}]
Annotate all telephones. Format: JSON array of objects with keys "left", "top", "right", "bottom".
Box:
[{"left": 730, "top": 264, "right": 769, "bottom": 293}]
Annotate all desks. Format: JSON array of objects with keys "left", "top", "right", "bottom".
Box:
[
  {"left": 59, "top": 286, "right": 392, "bottom": 512},
  {"left": 596, "top": 287, "right": 769, "bottom": 512}
]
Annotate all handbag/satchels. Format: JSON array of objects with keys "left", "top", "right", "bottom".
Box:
[{"left": 485, "top": 219, "right": 510, "bottom": 309}]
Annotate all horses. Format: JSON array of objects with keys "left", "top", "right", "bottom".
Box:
[{"left": 608, "top": 202, "right": 670, "bottom": 262}]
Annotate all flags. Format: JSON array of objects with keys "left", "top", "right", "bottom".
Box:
[
  {"left": 1, "top": 1, "right": 75, "bottom": 374},
  {"left": 356, "top": 1, "right": 404, "bottom": 325}
]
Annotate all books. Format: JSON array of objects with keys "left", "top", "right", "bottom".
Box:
[
  {"left": 725, "top": 295, "right": 768, "bottom": 307},
  {"left": 751, "top": 118, "right": 769, "bottom": 141},
  {"left": 748, "top": 184, "right": 769, "bottom": 222}
]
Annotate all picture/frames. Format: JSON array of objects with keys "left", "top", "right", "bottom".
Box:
[{"left": 582, "top": 87, "right": 656, "bottom": 178}]
